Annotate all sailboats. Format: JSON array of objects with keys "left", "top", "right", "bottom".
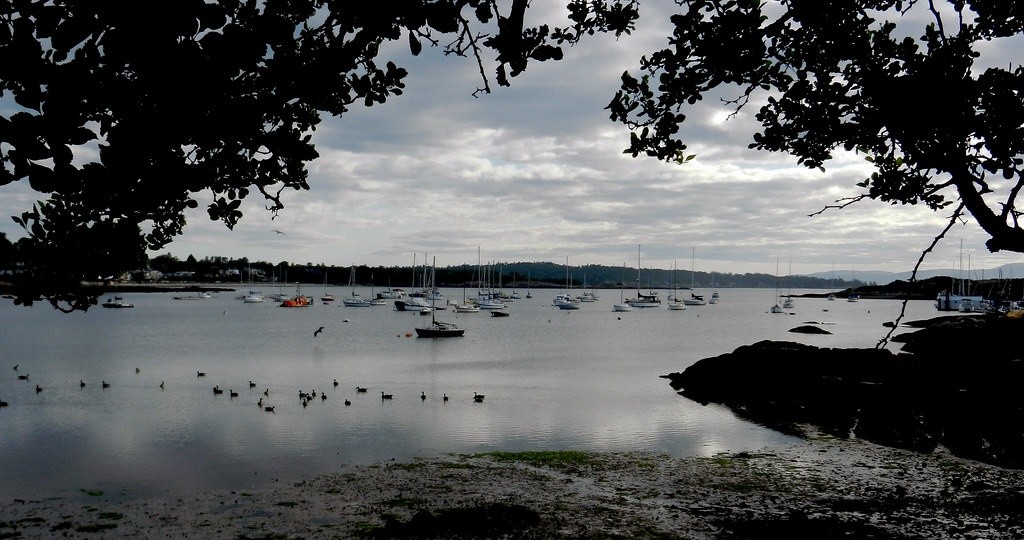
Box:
[
  {"left": 612, "top": 244, "right": 720, "bottom": 312},
  {"left": 828, "top": 264, "right": 836, "bottom": 301},
  {"left": 342, "top": 245, "right": 532, "bottom": 338},
  {"left": 846, "top": 274, "right": 859, "bottom": 302},
  {"left": 552, "top": 256, "right": 600, "bottom": 310},
  {"left": 770, "top": 257, "right": 795, "bottom": 313},
  {"left": 235, "top": 259, "right": 336, "bottom": 308},
  {"left": 936, "top": 238, "right": 983, "bottom": 310}
]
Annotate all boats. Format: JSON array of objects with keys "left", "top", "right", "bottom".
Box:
[
  {"left": 102, "top": 296, "right": 135, "bottom": 308},
  {"left": 173, "top": 290, "right": 220, "bottom": 301}
]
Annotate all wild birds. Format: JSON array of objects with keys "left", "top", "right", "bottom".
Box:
[
  {"left": 269, "top": 229, "right": 288, "bottom": 239},
  {"left": 0, "top": 365, "right": 484, "bottom": 413},
  {"left": 314, "top": 327, "right": 325, "bottom": 337}
]
